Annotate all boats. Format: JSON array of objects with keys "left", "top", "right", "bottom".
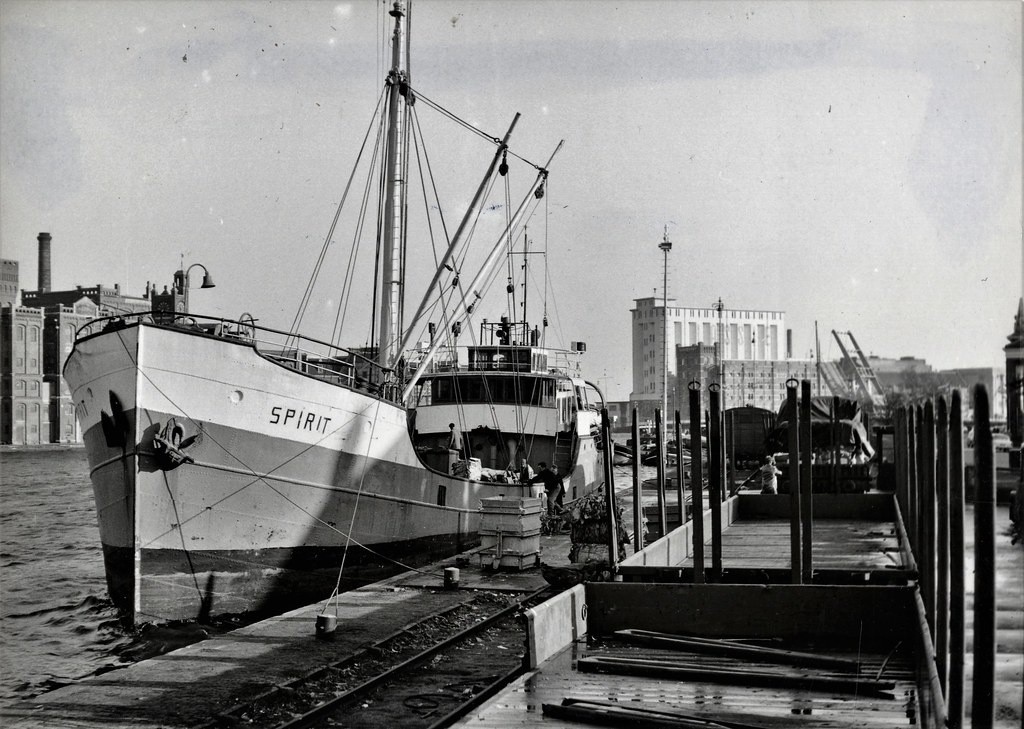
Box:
[
  {"left": 612, "top": 429, "right": 707, "bottom": 463},
  {"left": 407, "top": 315, "right": 616, "bottom": 525}
]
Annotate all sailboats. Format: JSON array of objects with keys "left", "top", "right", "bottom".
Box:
[{"left": 57, "top": 0, "right": 567, "bottom": 625}]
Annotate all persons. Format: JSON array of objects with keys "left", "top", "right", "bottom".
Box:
[
  {"left": 514, "top": 459, "right": 534, "bottom": 482},
  {"left": 550, "top": 464, "right": 566, "bottom": 508},
  {"left": 761, "top": 455, "right": 782, "bottom": 494},
  {"left": 446, "top": 423, "right": 465, "bottom": 452},
  {"left": 527, "top": 462, "right": 562, "bottom": 515}
]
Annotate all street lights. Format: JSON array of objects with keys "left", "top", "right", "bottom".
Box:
[
  {"left": 657, "top": 224, "right": 673, "bottom": 476},
  {"left": 178, "top": 261, "right": 215, "bottom": 325},
  {"left": 710, "top": 297, "right": 730, "bottom": 417}
]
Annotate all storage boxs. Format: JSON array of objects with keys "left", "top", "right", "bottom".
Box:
[{"left": 479, "top": 496, "right": 541, "bottom": 569}]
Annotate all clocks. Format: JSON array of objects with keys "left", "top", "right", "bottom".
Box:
[
  {"left": 177, "top": 301, "right": 185, "bottom": 312},
  {"left": 157, "top": 301, "right": 169, "bottom": 313}
]
